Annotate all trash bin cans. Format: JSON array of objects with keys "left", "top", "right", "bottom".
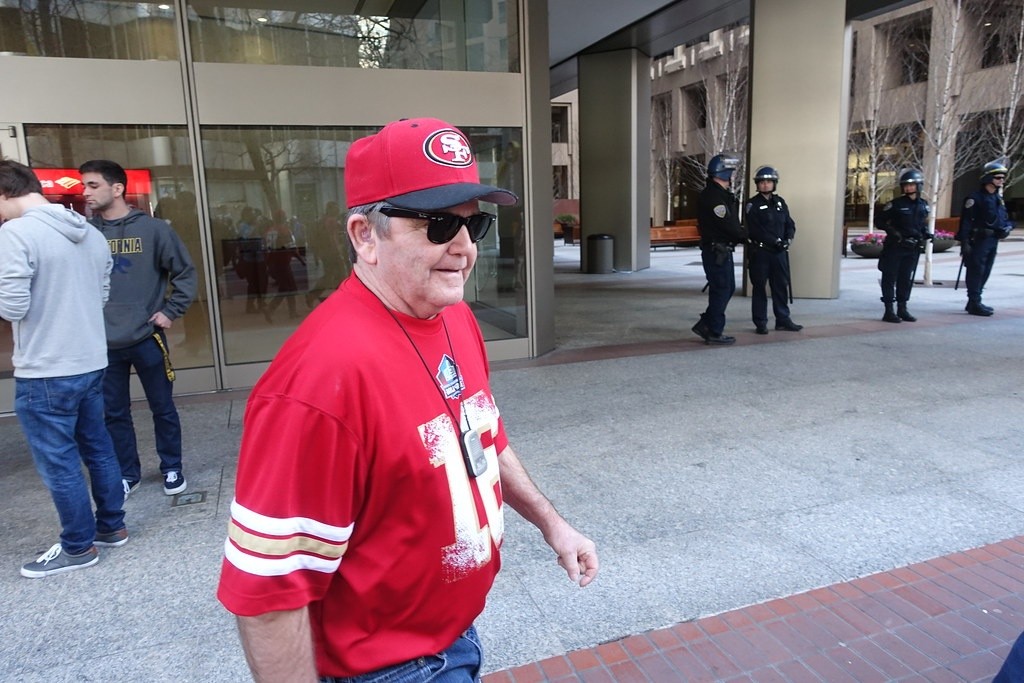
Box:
[{"left": 585, "top": 234, "right": 614, "bottom": 273}]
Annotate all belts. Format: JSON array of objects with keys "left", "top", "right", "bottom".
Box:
[{"left": 748, "top": 238, "right": 778, "bottom": 251}]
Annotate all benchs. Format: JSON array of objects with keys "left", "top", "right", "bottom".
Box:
[
  {"left": 572, "top": 224, "right": 580, "bottom": 245},
  {"left": 650, "top": 219, "right": 701, "bottom": 250},
  {"left": 553, "top": 221, "right": 564, "bottom": 238}
]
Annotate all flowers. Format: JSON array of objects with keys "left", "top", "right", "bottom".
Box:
[
  {"left": 851, "top": 234, "right": 886, "bottom": 246},
  {"left": 933, "top": 229, "right": 955, "bottom": 239}
]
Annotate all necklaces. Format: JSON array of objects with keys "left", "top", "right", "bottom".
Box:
[{"left": 355, "top": 274, "right": 488, "bottom": 477}]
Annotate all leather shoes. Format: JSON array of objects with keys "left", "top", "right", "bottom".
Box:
[
  {"left": 755, "top": 325, "right": 769, "bottom": 334},
  {"left": 705, "top": 334, "right": 735, "bottom": 345},
  {"left": 691, "top": 321, "right": 710, "bottom": 340},
  {"left": 775, "top": 317, "right": 803, "bottom": 332}
]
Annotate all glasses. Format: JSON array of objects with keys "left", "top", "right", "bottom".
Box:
[
  {"left": 992, "top": 175, "right": 1005, "bottom": 180},
  {"left": 360, "top": 207, "right": 497, "bottom": 245}
]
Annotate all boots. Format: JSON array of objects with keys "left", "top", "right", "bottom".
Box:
[
  {"left": 897, "top": 301, "right": 917, "bottom": 322},
  {"left": 966, "top": 296, "right": 993, "bottom": 312},
  {"left": 968, "top": 296, "right": 993, "bottom": 316},
  {"left": 882, "top": 301, "right": 902, "bottom": 323}
]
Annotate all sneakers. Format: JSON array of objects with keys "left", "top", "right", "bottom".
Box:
[
  {"left": 92, "top": 523, "right": 128, "bottom": 548},
  {"left": 20, "top": 542, "right": 100, "bottom": 579},
  {"left": 122, "top": 477, "right": 142, "bottom": 501},
  {"left": 161, "top": 468, "right": 187, "bottom": 496}
]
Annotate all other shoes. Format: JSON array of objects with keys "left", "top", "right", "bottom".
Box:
[{"left": 244, "top": 291, "right": 315, "bottom": 323}]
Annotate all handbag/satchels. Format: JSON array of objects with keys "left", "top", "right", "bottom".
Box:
[
  {"left": 236, "top": 260, "right": 247, "bottom": 279},
  {"left": 265, "top": 252, "right": 284, "bottom": 280}
]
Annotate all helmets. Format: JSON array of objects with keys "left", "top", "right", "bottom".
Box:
[
  {"left": 707, "top": 154, "right": 742, "bottom": 181},
  {"left": 752, "top": 167, "right": 778, "bottom": 194},
  {"left": 980, "top": 161, "right": 1007, "bottom": 182},
  {"left": 899, "top": 170, "right": 925, "bottom": 195}
]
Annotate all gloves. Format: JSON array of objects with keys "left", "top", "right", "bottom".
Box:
[
  {"left": 999, "top": 226, "right": 1011, "bottom": 237},
  {"left": 923, "top": 233, "right": 934, "bottom": 242},
  {"left": 894, "top": 231, "right": 902, "bottom": 243},
  {"left": 959, "top": 240, "right": 971, "bottom": 256}
]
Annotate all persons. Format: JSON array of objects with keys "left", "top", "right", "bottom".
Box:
[
  {"left": 875, "top": 170, "right": 930, "bottom": 323},
  {"left": 957, "top": 162, "right": 1016, "bottom": 317},
  {"left": 77, "top": 160, "right": 197, "bottom": 495},
  {"left": 305, "top": 200, "right": 339, "bottom": 310},
  {"left": 153, "top": 191, "right": 205, "bottom": 356},
  {"left": 217, "top": 200, "right": 302, "bottom": 321},
  {"left": 745, "top": 166, "right": 803, "bottom": 335},
  {"left": 0, "top": 159, "right": 129, "bottom": 578},
  {"left": 691, "top": 154, "right": 747, "bottom": 345},
  {"left": 216, "top": 117, "right": 599, "bottom": 683}
]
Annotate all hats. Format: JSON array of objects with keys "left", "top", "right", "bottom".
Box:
[{"left": 345, "top": 116, "right": 519, "bottom": 212}]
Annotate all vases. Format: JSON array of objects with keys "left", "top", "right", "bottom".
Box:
[
  {"left": 851, "top": 245, "right": 884, "bottom": 258},
  {"left": 932, "top": 238, "right": 956, "bottom": 252}
]
